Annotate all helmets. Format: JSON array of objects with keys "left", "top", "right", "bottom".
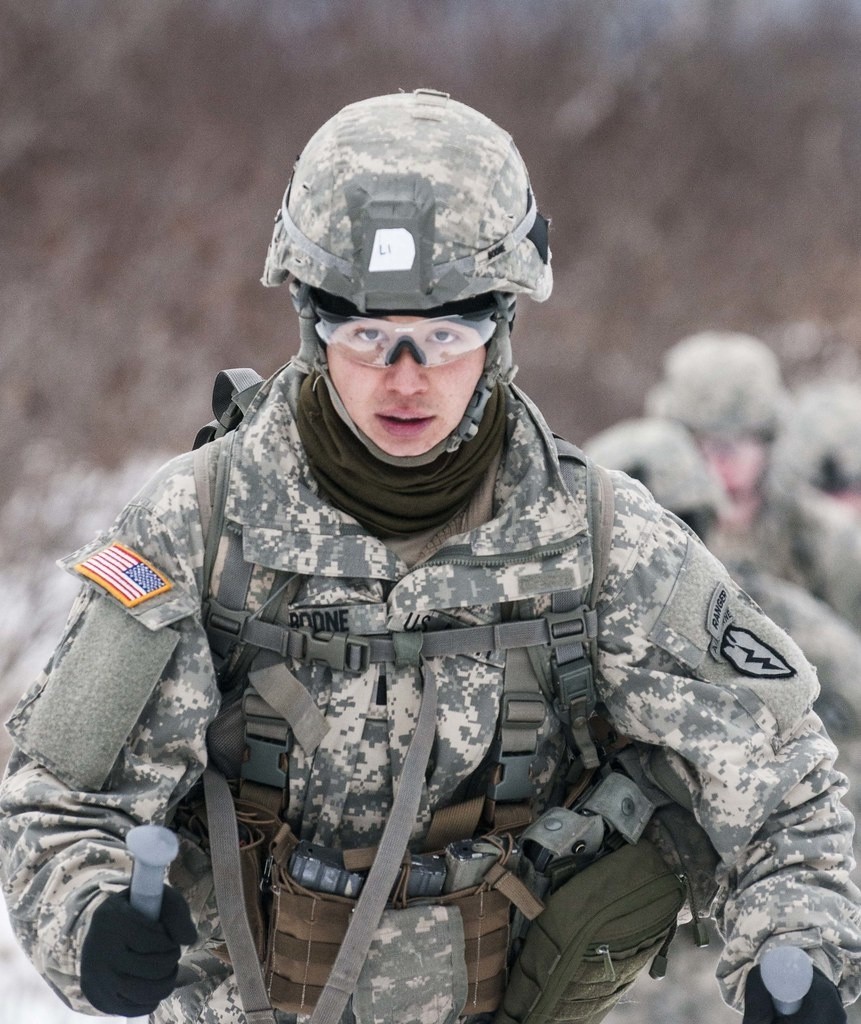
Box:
[
  {"left": 579, "top": 328, "right": 860, "bottom": 513},
  {"left": 260, "top": 87, "right": 552, "bottom": 313}
]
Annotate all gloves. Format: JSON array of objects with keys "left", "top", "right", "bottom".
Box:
[
  {"left": 80, "top": 883, "right": 198, "bottom": 1018},
  {"left": 742, "top": 963, "right": 847, "bottom": 1024}
]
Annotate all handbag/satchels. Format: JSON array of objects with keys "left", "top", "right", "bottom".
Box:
[{"left": 493, "top": 838, "right": 686, "bottom": 1024}]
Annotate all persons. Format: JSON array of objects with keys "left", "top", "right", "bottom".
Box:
[{"left": 1, "top": 89, "right": 861, "bottom": 1024}]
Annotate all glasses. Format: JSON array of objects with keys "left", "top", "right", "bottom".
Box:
[{"left": 302, "top": 299, "right": 512, "bottom": 367}]
